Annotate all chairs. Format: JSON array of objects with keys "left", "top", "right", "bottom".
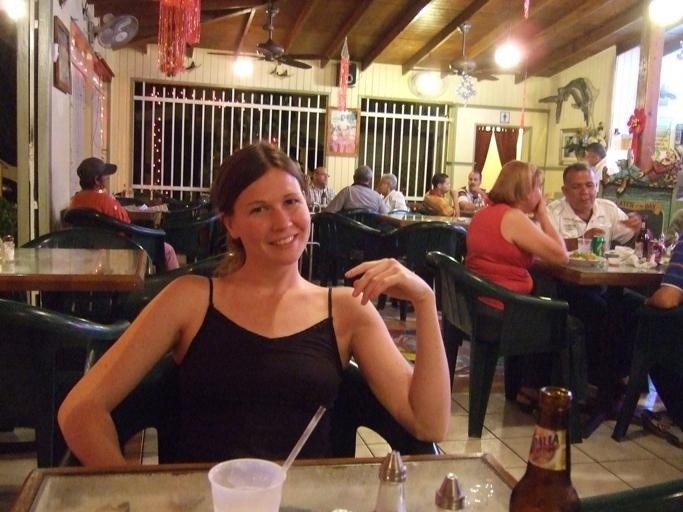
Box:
[{"left": 0, "top": 186, "right": 681, "bottom": 470}]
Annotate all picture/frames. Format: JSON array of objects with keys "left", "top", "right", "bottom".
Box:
[
  {"left": 324, "top": 107, "right": 359, "bottom": 157},
  {"left": 51, "top": 15, "right": 71, "bottom": 97}
]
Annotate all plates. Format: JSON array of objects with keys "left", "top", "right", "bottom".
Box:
[{"left": 568, "top": 257, "right": 600, "bottom": 266}]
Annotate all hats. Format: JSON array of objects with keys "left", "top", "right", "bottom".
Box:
[{"left": 76, "top": 157, "right": 116, "bottom": 178}]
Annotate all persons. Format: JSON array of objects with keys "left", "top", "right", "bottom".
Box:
[
  {"left": 635, "top": 226, "right": 682, "bottom": 433},
  {"left": 461, "top": 157, "right": 590, "bottom": 413},
  {"left": 421, "top": 171, "right": 460, "bottom": 218},
  {"left": 375, "top": 171, "right": 411, "bottom": 217},
  {"left": 65, "top": 156, "right": 182, "bottom": 275},
  {"left": 303, "top": 157, "right": 337, "bottom": 216},
  {"left": 324, "top": 159, "right": 389, "bottom": 227},
  {"left": 55, "top": 137, "right": 454, "bottom": 469},
  {"left": 456, "top": 169, "right": 489, "bottom": 212},
  {"left": 545, "top": 161, "right": 645, "bottom": 410}
]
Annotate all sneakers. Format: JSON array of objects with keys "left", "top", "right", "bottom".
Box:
[{"left": 639, "top": 407, "right": 683, "bottom": 448}]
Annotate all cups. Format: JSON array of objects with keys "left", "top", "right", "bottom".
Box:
[
  {"left": 204, "top": 458, "right": 287, "bottom": 512},
  {"left": 607, "top": 253, "right": 620, "bottom": 266},
  {"left": 596, "top": 224, "right": 611, "bottom": 251},
  {"left": 576, "top": 238, "right": 591, "bottom": 252}
]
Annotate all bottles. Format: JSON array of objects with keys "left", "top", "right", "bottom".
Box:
[
  {"left": 433, "top": 472, "right": 465, "bottom": 512},
  {"left": 2, "top": 235, "right": 15, "bottom": 264},
  {"left": 376, "top": 451, "right": 408, "bottom": 512},
  {"left": 121, "top": 182, "right": 134, "bottom": 198},
  {"left": 320, "top": 188, "right": 327, "bottom": 205},
  {"left": 508, "top": 385, "right": 580, "bottom": 512},
  {"left": 634, "top": 215, "right": 650, "bottom": 261}
]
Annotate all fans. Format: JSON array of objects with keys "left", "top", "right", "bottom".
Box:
[
  {"left": 410, "top": 23, "right": 521, "bottom": 85},
  {"left": 87, "top": 12, "right": 140, "bottom": 51},
  {"left": 206, "top": 7, "right": 313, "bottom": 71}
]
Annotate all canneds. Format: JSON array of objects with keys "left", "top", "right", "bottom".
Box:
[{"left": 592, "top": 235, "right": 607, "bottom": 257}]
